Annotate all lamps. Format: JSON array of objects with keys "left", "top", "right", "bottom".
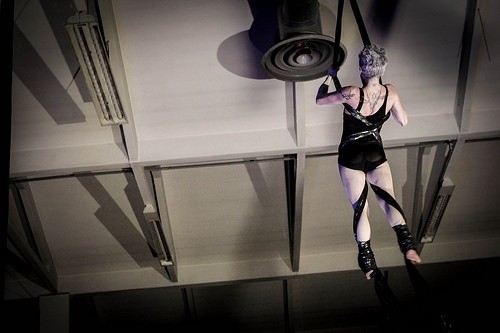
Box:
[
  {"left": 420, "top": 177, "right": 455, "bottom": 243},
  {"left": 260, "top": 29, "right": 348, "bottom": 83},
  {"left": 143, "top": 203, "right": 176, "bottom": 267},
  {"left": 64, "top": 10, "right": 130, "bottom": 127}
]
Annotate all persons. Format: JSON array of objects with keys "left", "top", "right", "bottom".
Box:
[{"left": 315, "top": 42, "right": 422, "bottom": 280}]
